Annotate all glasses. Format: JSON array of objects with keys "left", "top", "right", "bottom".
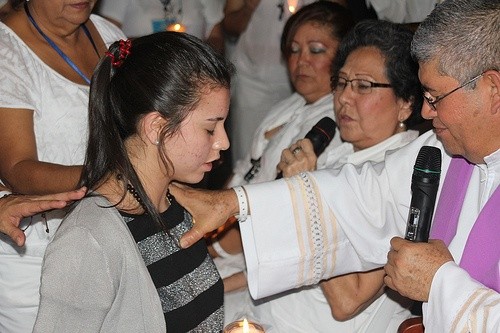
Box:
[
  {"left": 415, "top": 68, "right": 499, "bottom": 112},
  {"left": 329, "top": 75, "right": 394, "bottom": 95}
]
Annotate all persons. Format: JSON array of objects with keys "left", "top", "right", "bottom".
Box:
[
  {"left": 168, "top": 0, "right": 499, "bottom": 333},
  {"left": 0, "top": 1, "right": 442, "bottom": 333},
  {"left": 32, "top": 31, "right": 235, "bottom": 333}
]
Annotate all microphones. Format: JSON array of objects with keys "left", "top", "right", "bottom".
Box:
[
  {"left": 274, "top": 117, "right": 337, "bottom": 180},
  {"left": 406, "top": 146, "right": 441, "bottom": 243}
]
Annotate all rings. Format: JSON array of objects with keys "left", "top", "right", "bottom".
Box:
[{"left": 291, "top": 147, "right": 303, "bottom": 154}]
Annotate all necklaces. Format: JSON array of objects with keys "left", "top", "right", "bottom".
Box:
[{"left": 110, "top": 167, "right": 174, "bottom": 215}]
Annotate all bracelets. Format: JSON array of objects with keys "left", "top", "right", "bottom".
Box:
[
  {"left": 232, "top": 184, "right": 249, "bottom": 222},
  {"left": 0, "top": 193, "right": 49, "bottom": 234}
]
